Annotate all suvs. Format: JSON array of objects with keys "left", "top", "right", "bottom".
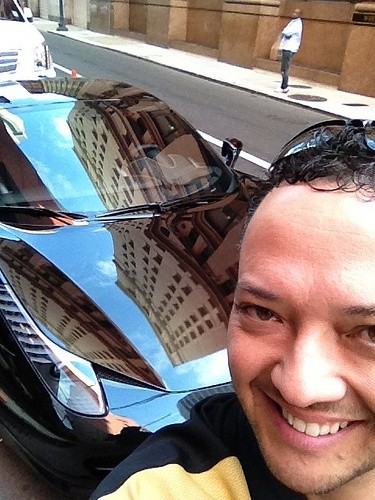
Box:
[{"left": 0, "top": 0, "right": 56, "bottom": 83}]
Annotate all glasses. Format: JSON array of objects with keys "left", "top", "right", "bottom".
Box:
[{"left": 268, "top": 121, "right": 375, "bottom": 170}]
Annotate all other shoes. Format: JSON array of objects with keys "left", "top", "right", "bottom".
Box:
[{"left": 277, "top": 86, "right": 289, "bottom": 94}]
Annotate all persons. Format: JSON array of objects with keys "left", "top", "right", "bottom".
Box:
[
  {"left": 88, "top": 118, "right": 375, "bottom": 498},
  {"left": 276, "top": 9, "right": 302, "bottom": 93}
]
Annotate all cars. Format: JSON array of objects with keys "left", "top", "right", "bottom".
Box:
[{"left": 0, "top": 74, "right": 271, "bottom": 492}]
